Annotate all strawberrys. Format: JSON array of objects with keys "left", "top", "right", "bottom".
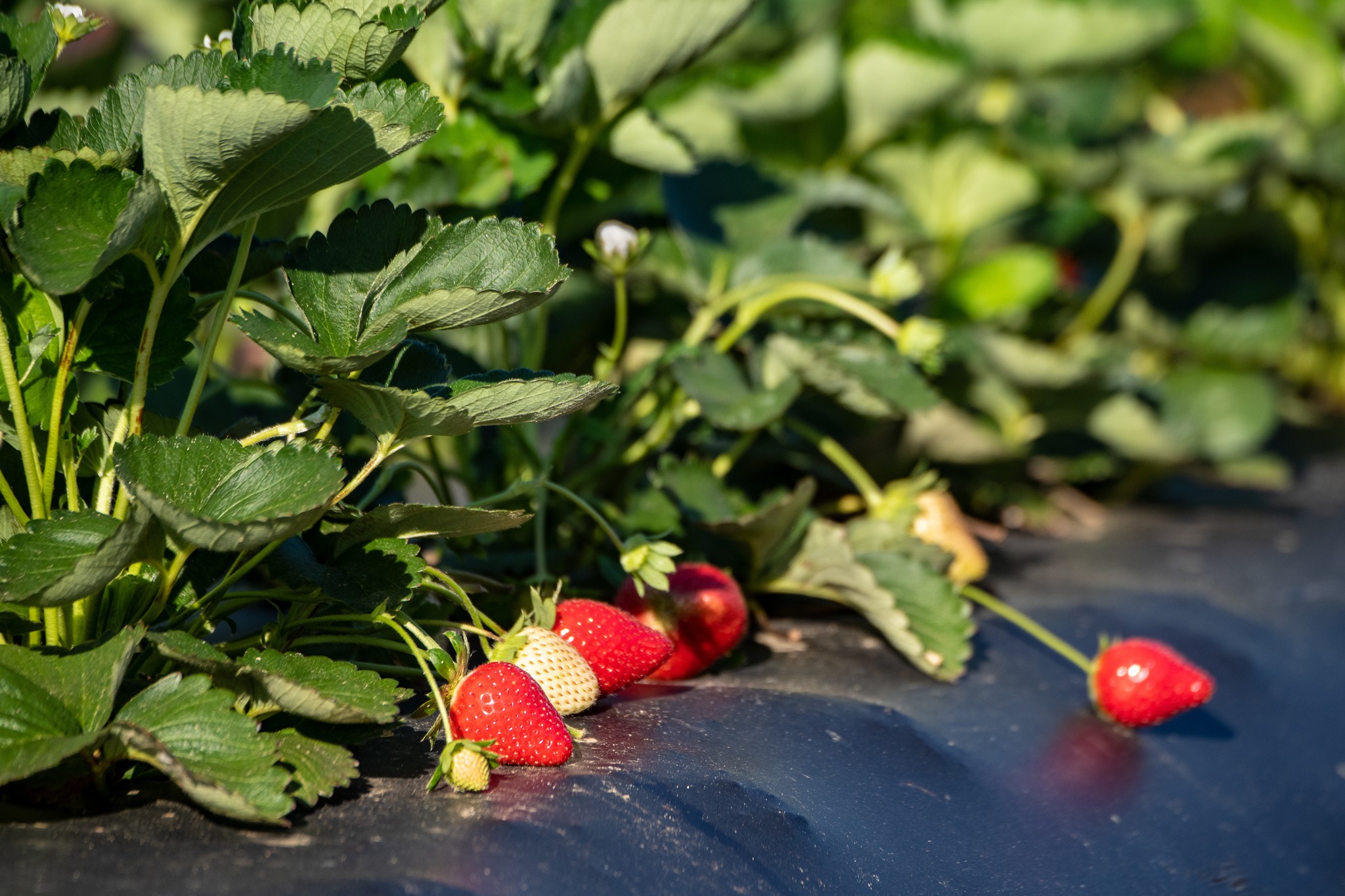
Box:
[
  {"left": 434, "top": 562, "right": 747, "bottom": 792},
  {"left": 1057, "top": 720, "right": 1136, "bottom": 799},
  {"left": 1083, "top": 632, "right": 1220, "bottom": 727}
]
